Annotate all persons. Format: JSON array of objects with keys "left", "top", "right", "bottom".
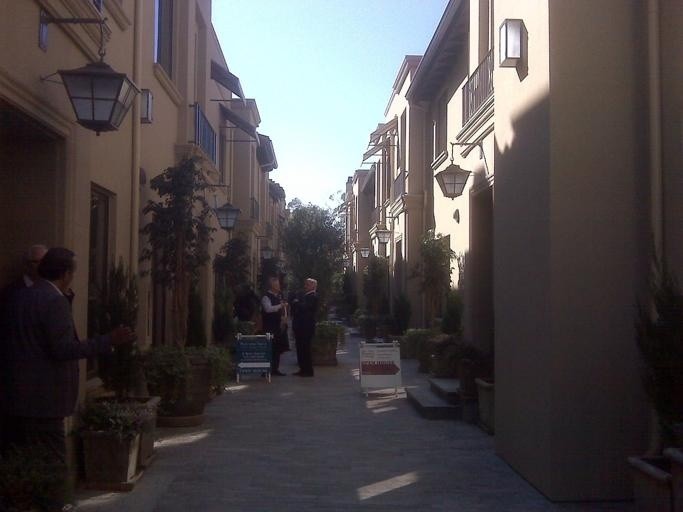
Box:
[
  {"left": 288, "top": 277, "right": 318, "bottom": 377},
  {"left": 259, "top": 276, "right": 292, "bottom": 377},
  {"left": 0, "top": 242, "right": 74, "bottom": 338},
  {"left": 0, "top": 247, "right": 137, "bottom": 511}
]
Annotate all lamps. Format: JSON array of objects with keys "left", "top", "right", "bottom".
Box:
[
  {"left": 432, "top": 138, "right": 485, "bottom": 201},
  {"left": 498, "top": 18, "right": 525, "bottom": 69},
  {"left": 256, "top": 235, "right": 274, "bottom": 261},
  {"left": 200, "top": 181, "right": 240, "bottom": 231},
  {"left": 35, "top": 10, "right": 142, "bottom": 137},
  {"left": 374, "top": 215, "right": 400, "bottom": 243}
]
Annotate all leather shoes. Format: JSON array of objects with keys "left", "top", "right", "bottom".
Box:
[
  {"left": 293, "top": 369, "right": 314, "bottom": 377},
  {"left": 262, "top": 368, "right": 286, "bottom": 377}
]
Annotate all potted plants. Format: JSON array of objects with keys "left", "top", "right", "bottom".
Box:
[
  {"left": 310, "top": 320, "right": 346, "bottom": 368},
  {"left": 411, "top": 222, "right": 467, "bottom": 377},
  {"left": 357, "top": 311, "right": 393, "bottom": 337},
  {"left": 623, "top": 233, "right": 683, "bottom": 510}
]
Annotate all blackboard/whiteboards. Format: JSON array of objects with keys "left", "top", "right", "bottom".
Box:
[{"left": 233, "top": 339, "right": 274, "bottom": 374}]
